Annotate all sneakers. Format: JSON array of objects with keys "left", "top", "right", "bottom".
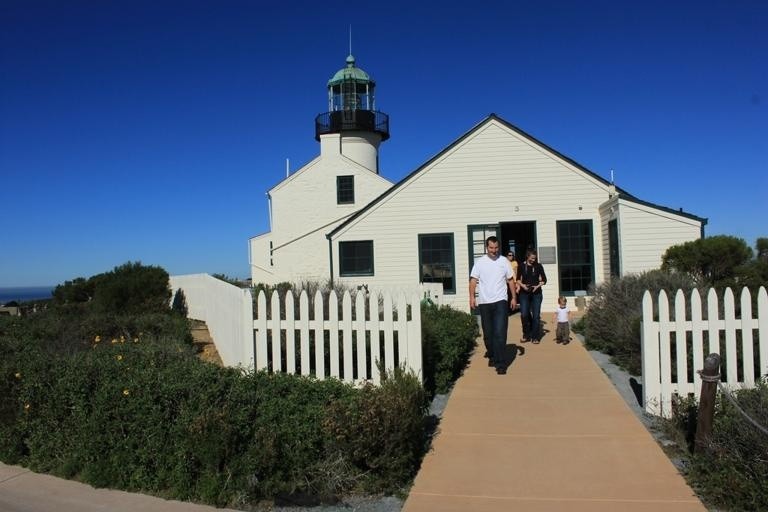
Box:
[
  {"left": 557, "top": 340, "right": 569, "bottom": 345},
  {"left": 489, "top": 357, "right": 506, "bottom": 374},
  {"left": 520, "top": 335, "right": 540, "bottom": 344}
]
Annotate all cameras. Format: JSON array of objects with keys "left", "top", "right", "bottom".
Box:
[{"left": 526, "top": 284, "right": 534, "bottom": 292}]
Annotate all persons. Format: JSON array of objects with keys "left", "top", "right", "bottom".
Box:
[
  {"left": 516, "top": 250, "right": 549, "bottom": 345},
  {"left": 469, "top": 236, "right": 518, "bottom": 375},
  {"left": 504, "top": 250, "right": 519, "bottom": 316},
  {"left": 551, "top": 295, "right": 575, "bottom": 345}
]
